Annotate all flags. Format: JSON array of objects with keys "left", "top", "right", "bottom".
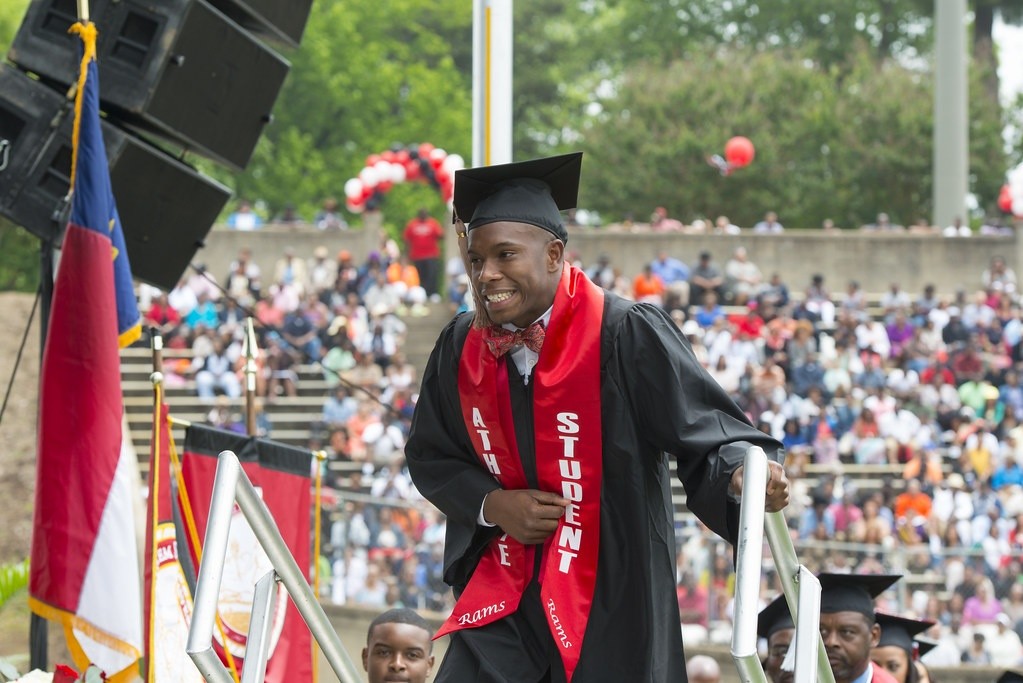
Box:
[{"left": 29, "top": 22, "right": 318, "bottom": 683}]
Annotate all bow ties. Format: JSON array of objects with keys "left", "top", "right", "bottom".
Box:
[{"left": 486, "top": 319, "right": 545, "bottom": 359}]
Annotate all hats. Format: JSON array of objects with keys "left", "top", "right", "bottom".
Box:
[
  {"left": 452, "top": 151, "right": 584, "bottom": 331},
  {"left": 816, "top": 572, "right": 903, "bottom": 621},
  {"left": 911, "top": 638, "right": 938, "bottom": 661},
  {"left": 757, "top": 592, "right": 795, "bottom": 639},
  {"left": 872, "top": 612, "right": 936, "bottom": 654}
]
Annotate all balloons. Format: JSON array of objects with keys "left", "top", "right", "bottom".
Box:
[
  {"left": 725, "top": 136, "right": 754, "bottom": 166},
  {"left": 343, "top": 141, "right": 467, "bottom": 214},
  {"left": 997, "top": 165, "right": 1023, "bottom": 219}
]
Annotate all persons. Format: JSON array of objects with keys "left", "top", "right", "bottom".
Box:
[
  {"left": 404, "top": 207, "right": 444, "bottom": 296},
  {"left": 226, "top": 201, "right": 261, "bottom": 231},
  {"left": 314, "top": 197, "right": 347, "bottom": 232},
  {"left": 129, "top": 234, "right": 477, "bottom": 614},
  {"left": 274, "top": 202, "right": 305, "bottom": 227},
  {"left": 561, "top": 208, "right": 1023, "bottom": 683},
  {"left": 361, "top": 608, "right": 435, "bottom": 683},
  {"left": 403, "top": 153, "right": 788, "bottom": 683}
]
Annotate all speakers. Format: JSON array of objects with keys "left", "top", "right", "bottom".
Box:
[{"left": 0, "top": 0, "right": 313, "bottom": 295}]
